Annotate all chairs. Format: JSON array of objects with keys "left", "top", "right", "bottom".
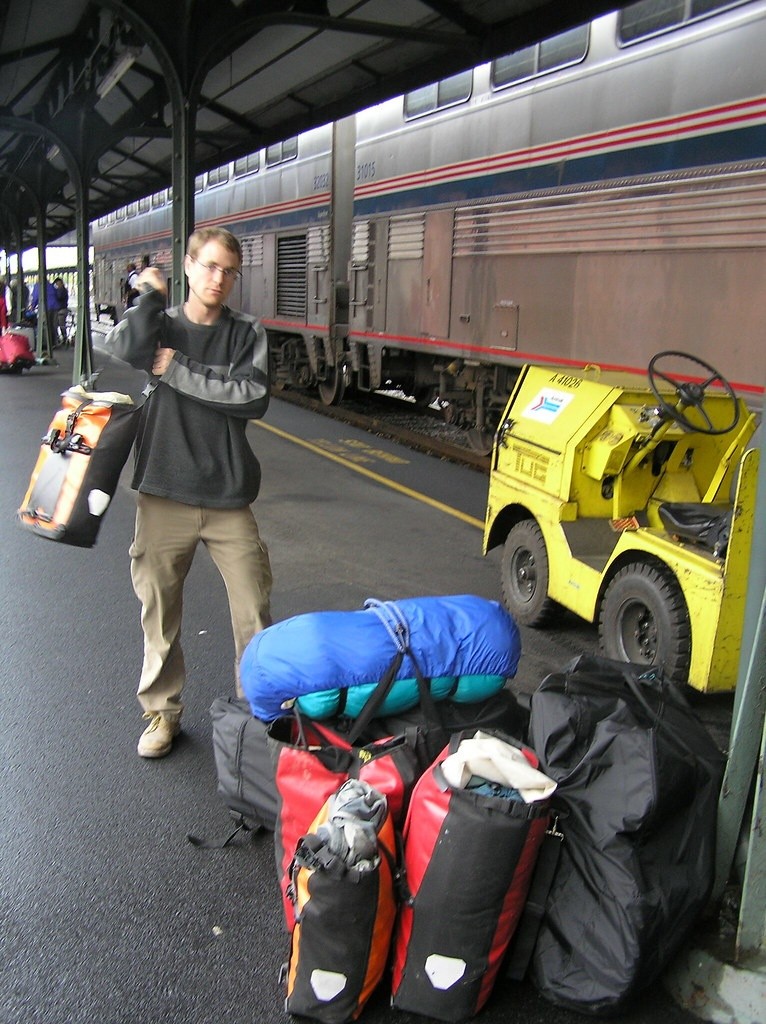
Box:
[{"left": 658, "top": 450, "right": 750, "bottom": 557}]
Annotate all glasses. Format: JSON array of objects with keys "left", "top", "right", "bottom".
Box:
[{"left": 194, "top": 257, "right": 243, "bottom": 282}]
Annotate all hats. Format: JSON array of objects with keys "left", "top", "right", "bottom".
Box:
[{"left": 9, "top": 279, "right": 17, "bottom": 289}]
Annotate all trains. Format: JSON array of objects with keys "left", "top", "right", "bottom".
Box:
[{"left": 90, "top": 2, "right": 766, "bottom": 440}]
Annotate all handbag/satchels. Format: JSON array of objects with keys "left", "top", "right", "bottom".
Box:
[
  {"left": 18, "top": 309, "right": 37, "bottom": 328},
  {"left": 17, "top": 383, "right": 139, "bottom": 549},
  {"left": 209, "top": 590, "right": 730, "bottom": 1024}
]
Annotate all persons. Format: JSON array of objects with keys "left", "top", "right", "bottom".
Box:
[
  {"left": 0, "top": 253, "right": 149, "bottom": 355},
  {"left": 102, "top": 226, "right": 272, "bottom": 757}
]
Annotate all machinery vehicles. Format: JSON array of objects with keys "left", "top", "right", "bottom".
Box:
[{"left": 478, "top": 347, "right": 755, "bottom": 695}]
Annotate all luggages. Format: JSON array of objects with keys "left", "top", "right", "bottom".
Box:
[{"left": 0, "top": 332, "right": 36, "bottom": 375}]
